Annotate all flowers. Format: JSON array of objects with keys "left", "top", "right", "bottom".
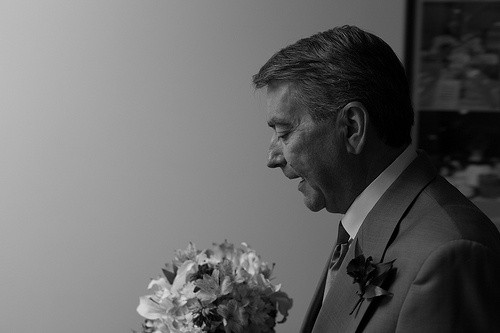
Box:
[
  {"left": 347, "top": 255, "right": 392, "bottom": 315},
  {"left": 135, "top": 238, "right": 293, "bottom": 333}
]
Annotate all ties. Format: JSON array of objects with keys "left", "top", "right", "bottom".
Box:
[{"left": 322, "top": 219, "right": 351, "bottom": 305}]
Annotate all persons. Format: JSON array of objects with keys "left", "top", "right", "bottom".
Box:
[{"left": 254, "top": 25, "right": 500, "bottom": 333}]
